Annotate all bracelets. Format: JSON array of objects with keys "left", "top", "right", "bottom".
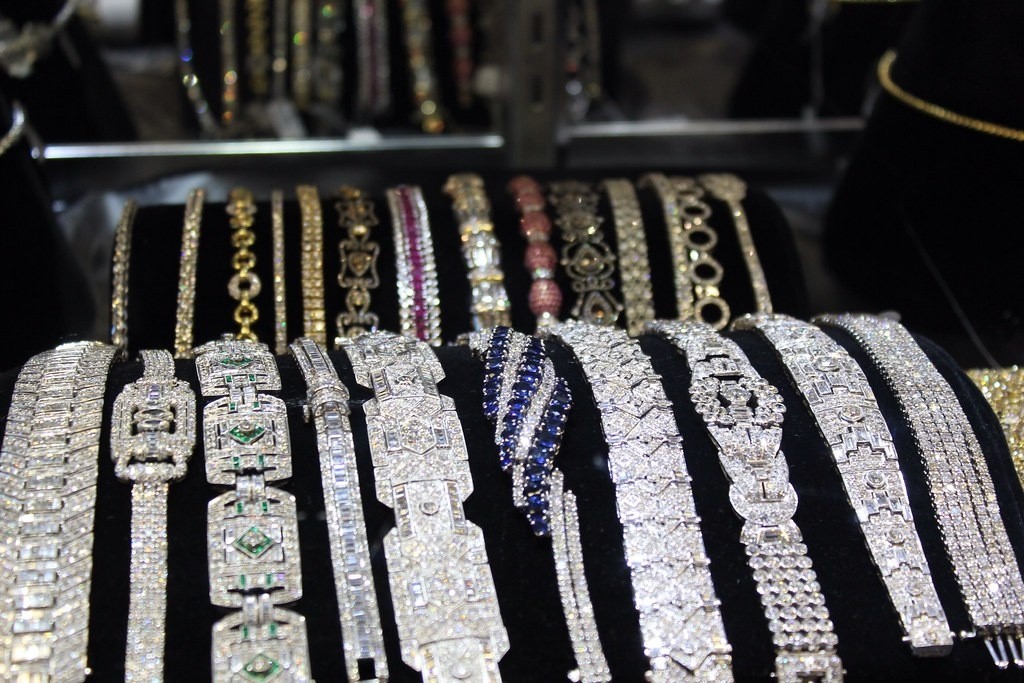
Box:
[
  {"left": 109, "top": 348, "right": 196, "bottom": 683},
  {"left": 965, "top": 365, "right": 1024, "bottom": 493},
  {"left": 697, "top": 170, "right": 773, "bottom": 315},
  {"left": 596, "top": 176, "right": 656, "bottom": 337},
  {"left": 111, "top": 197, "right": 137, "bottom": 359},
  {"left": 731, "top": 312, "right": 956, "bottom": 659},
  {"left": 333, "top": 328, "right": 512, "bottom": 683},
  {"left": 385, "top": 184, "right": 444, "bottom": 347},
  {"left": 191, "top": 332, "right": 317, "bottom": 683},
  {"left": 442, "top": 172, "right": 512, "bottom": 327},
  {"left": 646, "top": 318, "right": 849, "bottom": 683},
  {"left": 0, "top": 341, "right": 121, "bottom": 683},
  {"left": 0, "top": 100, "right": 26, "bottom": 156},
  {"left": 547, "top": 177, "right": 625, "bottom": 324},
  {"left": 289, "top": 335, "right": 389, "bottom": 683},
  {"left": 295, "top": 184, "right": 326, "bottom": 346},
  {"left": 225, "top": 186, "right": 259, "bottom": 343},
  {"left": 636, "top": 170, "right": 730, "bottom": 331},
  {"left": 52, "top": 0, "right": 75, "bottom": 26},
  {"left": 446, "top": 324, "right": 614, "bottom": 683},
  {"left": 271, "top": 188, "right": 288, "bottom": 354},
  {"left": 535, "top": 317, "right": 735, "bottom": 683},
  {"left": 332, "top": 183, "right": 379, "bottom": 337},
  {"left": 809, "top": 312, "right": 1024, "bottom": 671},
  {"left": 878, "top": 48, "right": 1024, "bottom": 142},
  {"left": 507, "top": 175, "right": 564, "bottom": 317},
  {"left": 174, "top": 188, "right": 204, "bottom": 360},
  {"left": 174, "top": 0, "right": 600, "bottom": 138}
]
